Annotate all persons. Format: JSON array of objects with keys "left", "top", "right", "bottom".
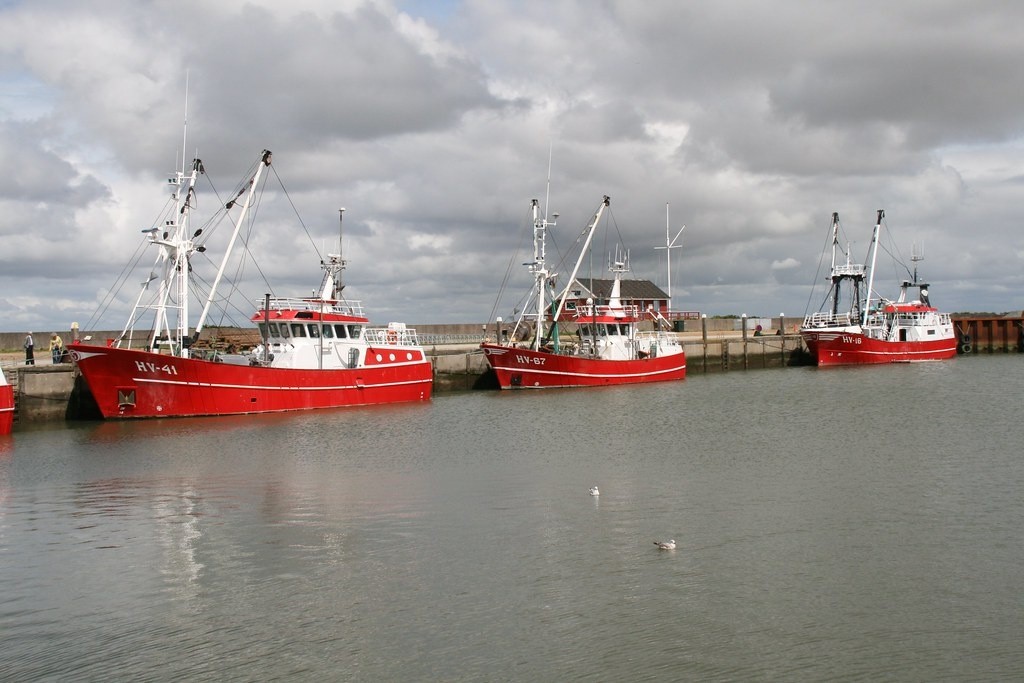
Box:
[
  {"left": 49, "top": 332, "right": 62, "bottom": 364},
  {"left": 23, "top": 331, "right": 34, "bottom": 365}
]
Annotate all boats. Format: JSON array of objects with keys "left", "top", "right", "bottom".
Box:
[
  {"left": 0, "top": 367, "right": 15, "bottom": 436},
  {"left": 799, "top": 209, "right": 957, "bottom": 366},
  {"left": 478, "top": 194, "right": 687, "bottom": 390},
  {"left": 64, "top": 148, "right": 435, "bottom": 420}
]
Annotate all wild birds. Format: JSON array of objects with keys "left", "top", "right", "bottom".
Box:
[
  {"left": 589, "top": 485, "right": 600, "bottom": 496},
  {"left": 653, "top": 539, "right": 676, "bottom": 550}
]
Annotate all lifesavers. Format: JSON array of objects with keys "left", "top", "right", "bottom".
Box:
[{"left": 387, "top": 331, "right": 398, "bottom": 345}]
